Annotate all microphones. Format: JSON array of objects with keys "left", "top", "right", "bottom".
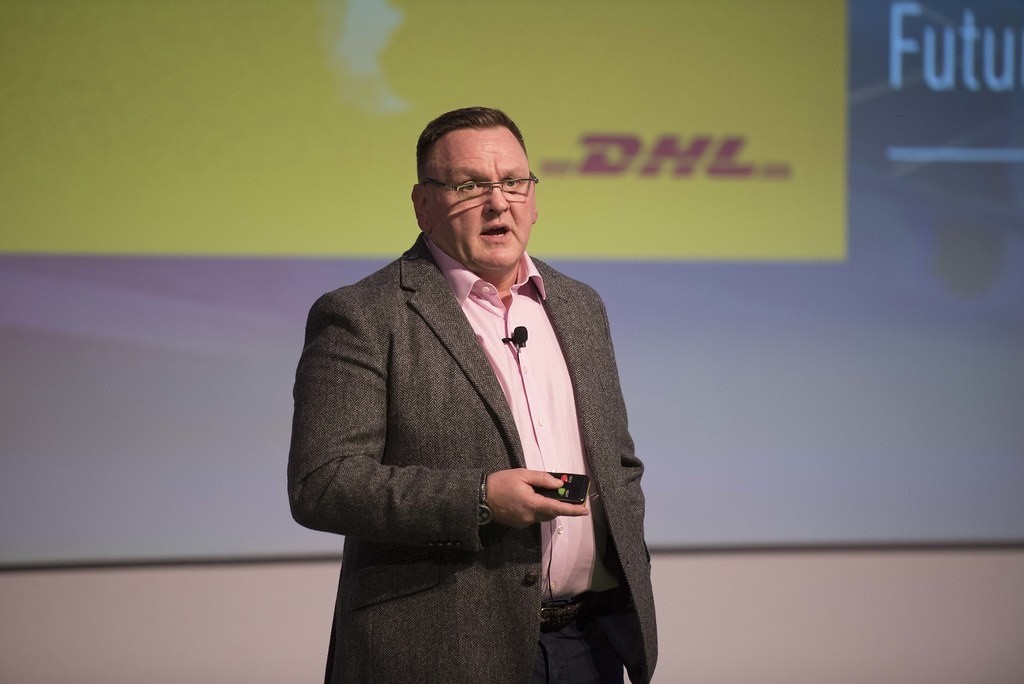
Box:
[{"left": 512, "top": 326, "right": 528, "bottom": 349}]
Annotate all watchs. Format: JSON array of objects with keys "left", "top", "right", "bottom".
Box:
[{"left": 477, "top": 473, "right": 493, "bottom": 526}]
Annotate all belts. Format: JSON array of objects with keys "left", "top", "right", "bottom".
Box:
[{"left": 540, "top": 588, "right": 633, "bottom": 633}]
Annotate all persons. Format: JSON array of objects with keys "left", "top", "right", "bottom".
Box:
[{"left": 286, "top": 105, "right": 658, "bottom": 684}]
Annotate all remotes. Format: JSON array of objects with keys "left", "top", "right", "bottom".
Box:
[{"left": 531, "top": 472, "right": 591, "bottom": 505}]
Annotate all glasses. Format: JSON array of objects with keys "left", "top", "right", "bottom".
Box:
[{"left": 421, "top": 169, "right": 540, "bottom": 206}]
